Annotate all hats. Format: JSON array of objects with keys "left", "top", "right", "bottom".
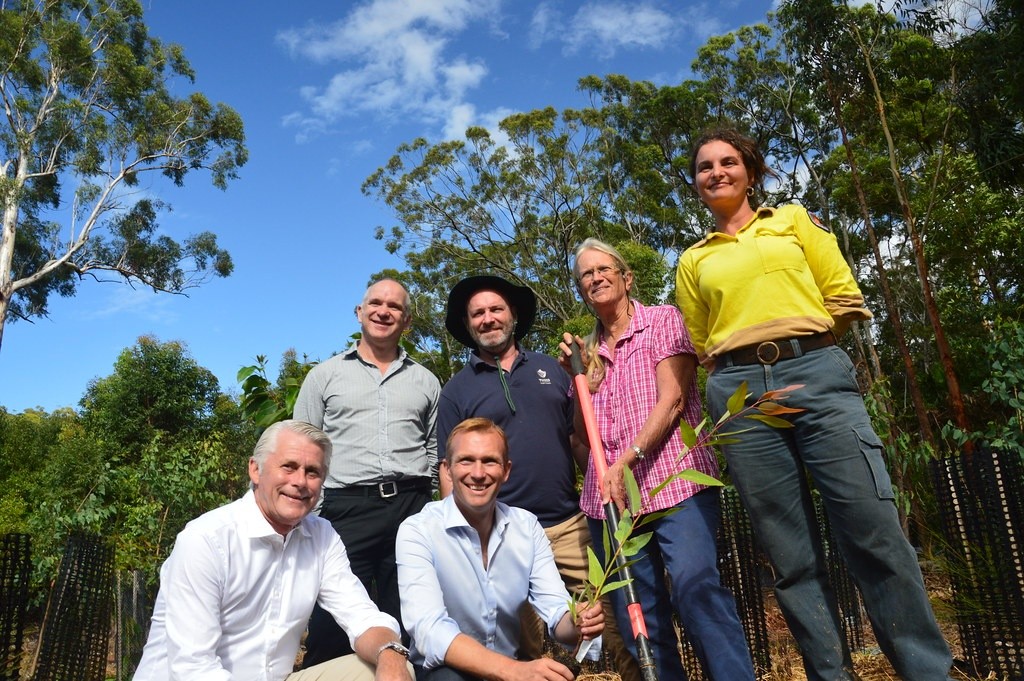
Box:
[{"left": 446, "top": 275, "right": 536, "bottom": 351}]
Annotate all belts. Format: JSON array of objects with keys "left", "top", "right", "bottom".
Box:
[
  {"left": 716, "top": 330, "right": 836, "bottom": 369},
  {"left": 324, "top": 477, "right": 428, "bottom": 499}
]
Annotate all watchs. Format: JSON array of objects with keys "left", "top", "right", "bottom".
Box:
[
  {"left": 631, "top": 443, "right": 645, "bottom": 461},
  {"left": 377, "top": 641, "right": 411, "bottom": 662}
]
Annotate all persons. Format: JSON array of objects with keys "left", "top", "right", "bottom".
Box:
[
  {"left": 396, "top": 418, "right": 606, "bottom": 681},
  {"left": 439, "top": 274, "right": 646, "bottom": 681},
  {"left": 134, "top": 421, "right": 416, "bottom": 680},
  {"left": 674, "top": 127, "right": 957, "bottom": 680},
  {"left": 559, "top": 238, "right": 760, "bottom": 681},
  {"left": 294, "top": 278, "right": 442, "bottom": 675}
]
